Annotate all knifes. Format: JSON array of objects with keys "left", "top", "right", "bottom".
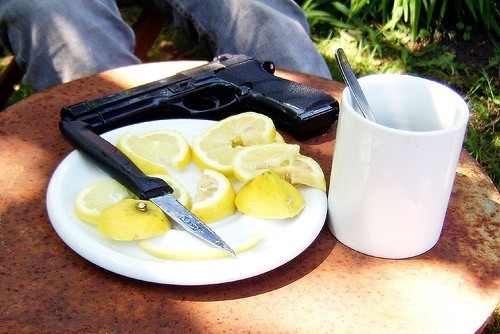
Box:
[{"left": 59, "top": 119, "right": 238, "bottom": 257}]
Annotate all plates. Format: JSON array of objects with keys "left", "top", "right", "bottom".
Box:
[{"left": 42, "top": 118, "right": 329, "bottom": 288}]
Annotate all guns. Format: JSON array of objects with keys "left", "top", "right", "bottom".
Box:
[{"left": 59, "top": 52, "right": 339, "bottom": 143}]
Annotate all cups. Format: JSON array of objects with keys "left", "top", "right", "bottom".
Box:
[{"left": 325, "top": 73, "right": 469, "bottom": 259}]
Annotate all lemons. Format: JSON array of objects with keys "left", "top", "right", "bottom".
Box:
[{"left": 75, "top": 111, "right": 327, "bottom": 239}]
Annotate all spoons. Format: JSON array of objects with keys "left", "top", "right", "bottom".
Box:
[{"left": 333, "top": 46, "right": 384, "bottom": 125}]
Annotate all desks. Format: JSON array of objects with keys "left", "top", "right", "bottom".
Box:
[{"left": 0, "top": 57, "right": 500, "bottom": 333}]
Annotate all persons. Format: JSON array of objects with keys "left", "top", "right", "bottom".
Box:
[{"left": 1, "top": 0, "right": 334, "bottom": 98}]
